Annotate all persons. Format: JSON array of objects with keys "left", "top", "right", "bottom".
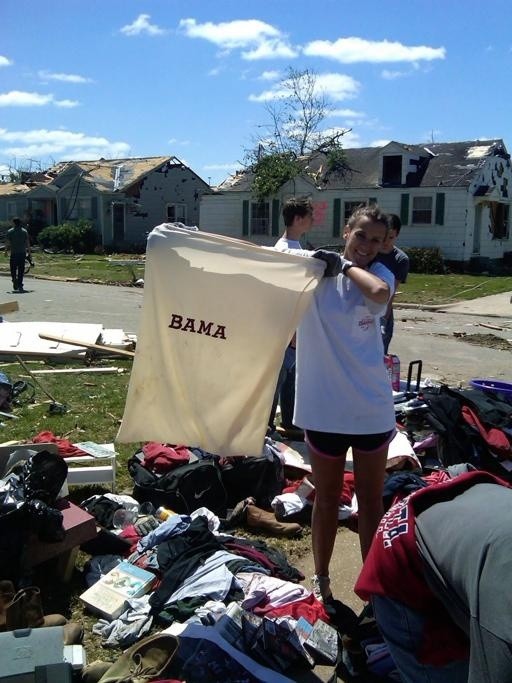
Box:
[
  {"left": 372, "top": 213, "right": 411, "bottom": 357},
  {"left": 3, "top": 217, "right": 32, "bottom": 292},
  {"left": 266, "top": 198, "right": 315, "bottom": 432},
  {"left": 353, "top": 471, "right": 512, "bottom": 683},
  {"left": 174, "top": 201, "right": 400, "bottom": 614}
]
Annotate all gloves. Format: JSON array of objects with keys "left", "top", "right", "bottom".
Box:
[{"left": 311, "top": 249, "right": 356, "bottom": 279}]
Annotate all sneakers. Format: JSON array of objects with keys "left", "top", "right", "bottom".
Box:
[
  {"left": 96, "top": 631, "right": 181, "bottom": 683},
  {"left": 310, "top": 571, "right": 333, "bottom": 602}
]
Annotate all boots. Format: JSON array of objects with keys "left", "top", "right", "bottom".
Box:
[
  {"left": 245, "top": 505, "right": 304, "bottom": 538},
  {"left": 0, "top": 579, "right": 85, "bottom": 645}
]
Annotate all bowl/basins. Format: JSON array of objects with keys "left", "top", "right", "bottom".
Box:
[{"left": 469, "top": 376, "right": 512, "bottom": 402}]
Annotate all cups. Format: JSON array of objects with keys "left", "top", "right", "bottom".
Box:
[
  {"left": 113, "top": 509, "right": 142, "bottom": 528},
  {"left": 138, "top": 502, "right": 157, "bottom": 517},
  {"left": 154, "top": 506, "right": 170, "bottom": 521}
]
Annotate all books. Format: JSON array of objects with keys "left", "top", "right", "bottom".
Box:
[{"left": 78, "top": 559, "right": 157, "bottom": 622}]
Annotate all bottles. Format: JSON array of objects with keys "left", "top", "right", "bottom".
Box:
[{"left": 386, "top": 353, "right": 400, "bottom": 394}]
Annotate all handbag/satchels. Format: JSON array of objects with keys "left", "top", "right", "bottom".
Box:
[{"left": 126, "top": 439, "right": 285, "bottom": 526}]
